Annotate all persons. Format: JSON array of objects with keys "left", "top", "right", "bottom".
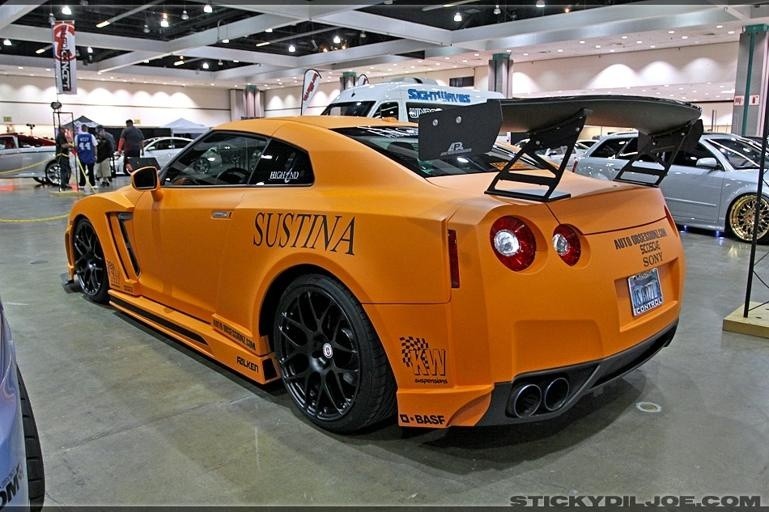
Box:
[
  {"left": 54, "top": 125, "right": 116, "bottom": 190},
  {"left": 118, "top": 119, "right": 144, "bottom": 176}
]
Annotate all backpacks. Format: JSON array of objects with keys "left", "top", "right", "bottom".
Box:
[{"left": 104, "top": 139, "right": 114, "bottom": 158}]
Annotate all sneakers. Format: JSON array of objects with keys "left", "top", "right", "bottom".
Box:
[
  {"left": 89, "top": 181, "right": 96, "bottom": 186},
  {"left": 60, "top": 185, "right": 72, "bottom": 189},
  {"left": 99, "top": 181, "right": 110, "bottom": 188},
  {"left": 79, "top": 182, "right": 86, "bottom": 186}
]
